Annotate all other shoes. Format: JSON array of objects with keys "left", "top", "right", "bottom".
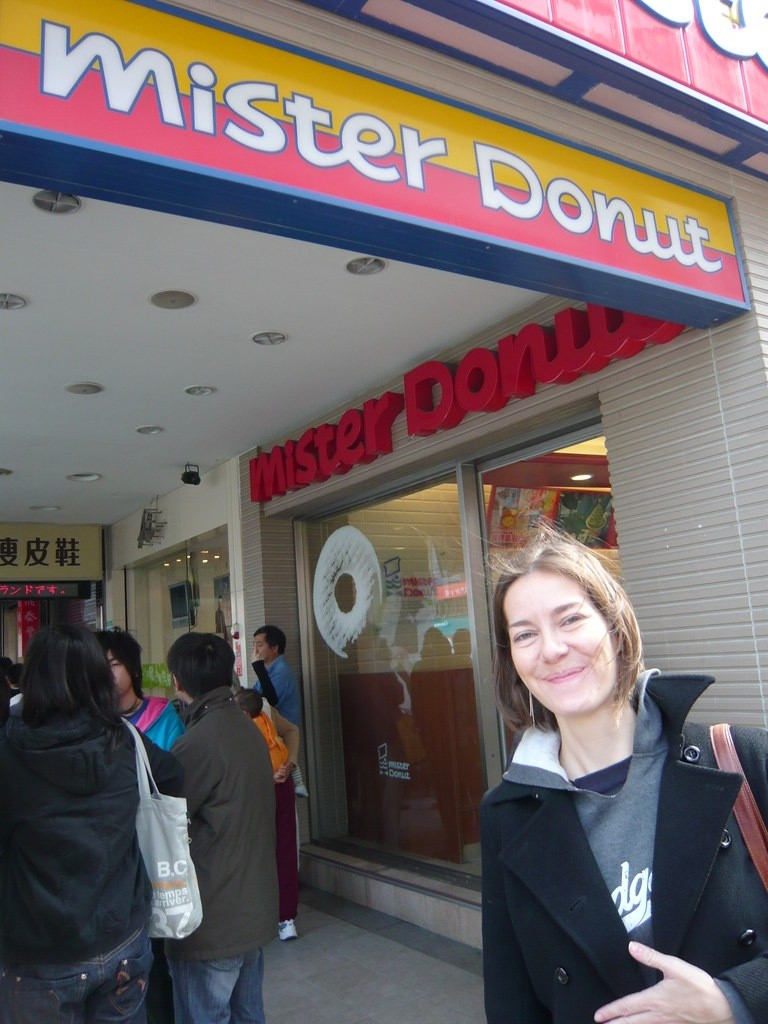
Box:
[{"left": 295, "top": 786, "right": 308, "bottom": 799}]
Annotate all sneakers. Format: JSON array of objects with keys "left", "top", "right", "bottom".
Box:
[{"left": 278, "top": 919, "right": 297, "bottom": 941}]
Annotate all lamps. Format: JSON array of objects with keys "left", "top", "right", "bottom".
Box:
[{"left": 179, "top": 462, "right": 201, "bottom": 485}]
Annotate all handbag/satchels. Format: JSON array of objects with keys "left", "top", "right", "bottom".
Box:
[{"left": 120, "top": 715, "right": 203, "bottom": 939}]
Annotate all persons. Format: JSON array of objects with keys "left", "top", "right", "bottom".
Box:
[
  {"left": 341, "top": 619, "right": 480, "bottom": 861},
  {"left": 91, "top": 624, "right": 188, "bottom": 753},
  {"left": 130, "top": 632, "right": 278, "bottom": 1024},
  {"left": 235, "top": 690, "right": 308, "bottom": 798},
  {"left": 0, "top": 614, "right": 187, "bottom": 1024},
  {"left": 479, "top": 528, "right": 768, "bottom": 1024},
  {"left": 228, "top": 669, "right": 312, "bottom": 940},
  {"left": 252, "top": 626, "right": 304, "bottom": 872}
]
